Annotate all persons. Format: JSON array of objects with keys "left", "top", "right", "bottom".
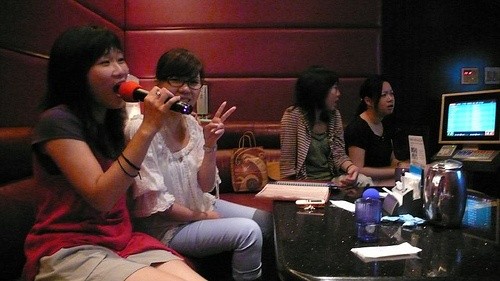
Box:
[
  {"left": 280, "top": 64, "right": 375, "bottom": 186},
  {"left": 343, "top": 74, "right": 410, "bottom": 179},
  {"left": 123, "top": 47, "right": 275, "bottom": 281},
  {"left": 21, "top": 25, "right": 208, "bottom": 281}
]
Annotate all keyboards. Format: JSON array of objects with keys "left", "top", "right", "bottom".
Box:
[{"left": 451, "top": 150, "right": 500, "bottom": 161}]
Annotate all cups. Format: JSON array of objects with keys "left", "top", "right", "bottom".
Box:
[
  {"left": 394, "top": 167, "right": 409, "bottom": 183},
  {"left": 354, "top": 198, "right": 382, "bottom": 242}
]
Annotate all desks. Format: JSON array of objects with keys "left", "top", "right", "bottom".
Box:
[{"left": 272, "top": 185, "right": 500, "bottom": 281}]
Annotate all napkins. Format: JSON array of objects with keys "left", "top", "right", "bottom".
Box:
[
  {"left": 329, "top": 200, "right": 356, "bottom": 214},
  {"left": 350, "top": 242, "right": 422, "bottom": 259}
]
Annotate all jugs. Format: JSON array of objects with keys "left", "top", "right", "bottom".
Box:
[{"left": 423, "top": 158, "right": 465, "bottom": 230}]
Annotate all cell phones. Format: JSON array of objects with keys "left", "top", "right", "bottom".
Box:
[
  {"left": 296, "top": 209, "right": 326, "bottom": 217},
  {"left": 295, "top": 199, "right": 325, "bottom": 208}
]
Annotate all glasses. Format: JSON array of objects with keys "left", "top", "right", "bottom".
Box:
[{"left": 160, "top": 74, "right": 204, "bottom": 90}]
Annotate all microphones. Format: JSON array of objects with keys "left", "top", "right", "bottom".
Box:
[
  {"left": 364, "top": 189, "right": 388, "bottom": 200},
  {"left": 119, "top": 81, "right": 193, "bottom": 114}
]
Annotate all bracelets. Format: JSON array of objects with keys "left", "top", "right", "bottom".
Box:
[
  {"left": 346, "top": 163, "right": 355, "bottom": 173},
  {"left": 397, "top": 161, "right": 402, "bottom": 166},
  {"left": 203, "top": 144, "right": 217, "bottom": 152},
  {"left": 120, "top": 153, "right": 140, "bottom": 171},
  {"left": 117, "top": 158, "right": 142, "bottom": 180}
]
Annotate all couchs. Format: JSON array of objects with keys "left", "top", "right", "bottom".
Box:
[{"left": 0, "top": 121, "right": 280, "bottom": 281}]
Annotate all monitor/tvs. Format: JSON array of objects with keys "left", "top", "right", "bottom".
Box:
[{"left": 438, "top": 90, "right": 500, "bottom": 149}]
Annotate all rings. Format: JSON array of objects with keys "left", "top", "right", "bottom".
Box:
[{"left": 156, "top": 91, "right": 161, "bottom": 96}]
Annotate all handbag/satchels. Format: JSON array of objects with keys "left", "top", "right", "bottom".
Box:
[{"left": 230, "top": 132, "right": 267, "bottom": 193}]
